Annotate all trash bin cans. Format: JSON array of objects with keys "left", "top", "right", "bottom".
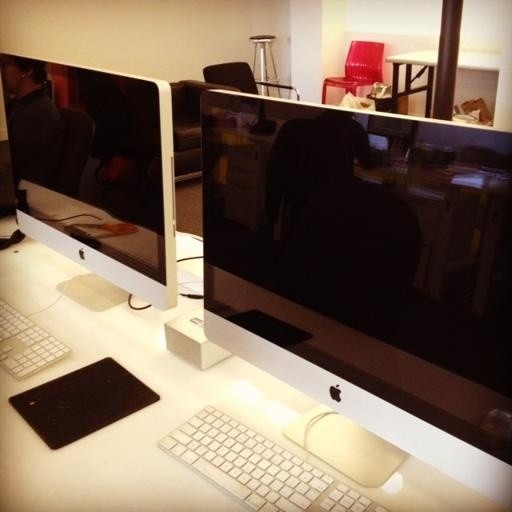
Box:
[
  {"left": 451, "top": 112, "right": 479, "bottom": 124},
  {"left": 367, "top": 94, "right": 392, "bottom": 112}
]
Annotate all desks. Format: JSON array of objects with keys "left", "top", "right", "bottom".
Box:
[
  {"left": 384, "top": 52, "right": 501, "bottom": 115},
  {"left": 1, "top": 229, "right": 512, "bottom": 512}
]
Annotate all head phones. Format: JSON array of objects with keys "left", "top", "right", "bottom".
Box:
[{"left": 31, "top": 60, "right": 48, "bottom": 85}]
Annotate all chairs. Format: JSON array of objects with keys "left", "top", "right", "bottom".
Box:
[
  {"left": 322, "top": 41, "right": 385, "bottom": 104},
  {"left": 168, "top": 62, "right": 300, "bottom": 185}
]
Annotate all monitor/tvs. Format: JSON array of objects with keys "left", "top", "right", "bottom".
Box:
[
  {"left": 201, "top": 86, "right": 512, "bottom": 507},
  {"left": 367, "top": 132, "right": 390, "bottom": 151},
  {"left": 0, "top": 47, "right": 180, "bottom": 315}
]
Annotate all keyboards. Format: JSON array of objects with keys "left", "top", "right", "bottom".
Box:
[
  {"left": 0, "top": 296, "right": 72, "bottom": 382},
  {"left": 157, "top": 402, "right": 392, "bottom": 512}
]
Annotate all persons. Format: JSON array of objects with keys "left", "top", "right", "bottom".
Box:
[
  {"left": 263, "top": 109, "right": 377, "bottom": 258},
  {"left": 0, "top": 54, "right": 69, "bottom": 194}
]
